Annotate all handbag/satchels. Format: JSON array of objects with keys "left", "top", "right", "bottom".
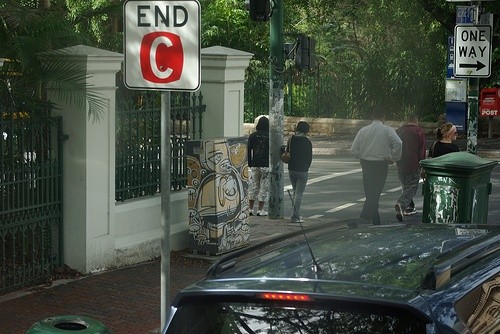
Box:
[{"left": 281, "top": 135, "right": 293, "bottom": 163}]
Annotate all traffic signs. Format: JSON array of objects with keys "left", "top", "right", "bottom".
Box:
[{"left": 452, "top": 23, "right": 493, "bottom": 79}]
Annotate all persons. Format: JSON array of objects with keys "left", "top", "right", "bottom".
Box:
[
  {"left": 284, "top": 121, "right": 312, "bottom": 223},
  {"left": 248, "top": 116, "right": 270, "bottom": 216},
  {"left": 350, "top": 106, "right": 402, "bottom": 225},
  {"left": 427, "top": 122, "right": 460, "bottom": 157},
  {"left": 394, "top": 114, "right": 426, "bottom": 220}
]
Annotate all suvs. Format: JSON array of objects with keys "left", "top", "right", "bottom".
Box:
[{"left": 159, "top": 217, "right": 500, "bottom": 334}]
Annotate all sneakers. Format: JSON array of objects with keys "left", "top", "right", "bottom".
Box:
[
  {"left": 395, "top": 203, "right": 403, "bottom": 221},
  {"left": 404, "top": 209, "right": 416, "bottom": 215}
]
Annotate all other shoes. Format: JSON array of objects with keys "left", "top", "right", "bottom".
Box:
[
  {"left": 299, "top": 216, "right": 303, "bottom": 218},
  {"left": 248, "top": 209, "right": 254, "bottom": 216},
  {"left": 296, "top": 220, "right": 305, "bottom": 222},
  {"left": 257, "top": 209, "right": 268, "bottom": 216}
]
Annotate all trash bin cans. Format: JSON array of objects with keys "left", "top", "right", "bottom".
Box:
[
  {"left": 26, "top": 314, "right": 111, "bottom": 334},
  {"left": 418, "top": 151, "right": 498, "bottom": 224}
]
